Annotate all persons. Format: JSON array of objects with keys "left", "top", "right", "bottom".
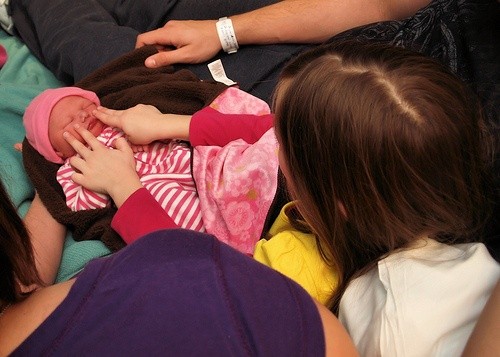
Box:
[
  {"left": 134, "top": 0, "right": 500, "bottom": 68},
  {"left": 21, "top": 82, "right": 210, "bottom": 233},
  {"left": 62, "top": 40, "right": 500, "bottom": 357},
  {"left": 0, "top": 177, "right": 364, "bottom": 357}
]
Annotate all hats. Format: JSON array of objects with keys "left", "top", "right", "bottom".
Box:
[{"left": 23, "top": 86, "right": 100, "bottom": 163}]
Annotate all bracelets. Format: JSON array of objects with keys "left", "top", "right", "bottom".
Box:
[{"left": 215, "top": 16, "right": 239, "bottom": 55}]
